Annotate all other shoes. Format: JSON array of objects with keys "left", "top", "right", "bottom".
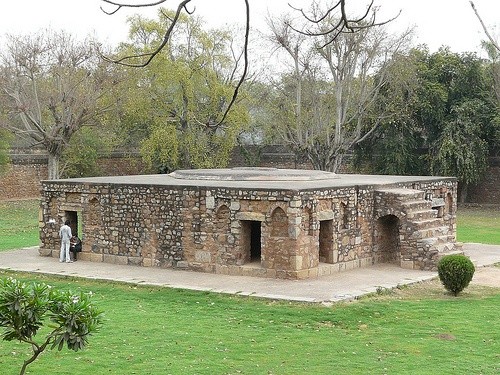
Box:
[
  {"left": 75, "top": 259, "right": 77, "bottom": 261},
  {"left": 68, "top": 261, "right": 73, "bottom": 263}
]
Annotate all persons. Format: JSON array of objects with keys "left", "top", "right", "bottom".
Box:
[
  {"left": 69, "top": 233, "right": 82, "bottom": 262},
  {"left": 58, "top": 220, "right": 74, "bottom": 263}
]
